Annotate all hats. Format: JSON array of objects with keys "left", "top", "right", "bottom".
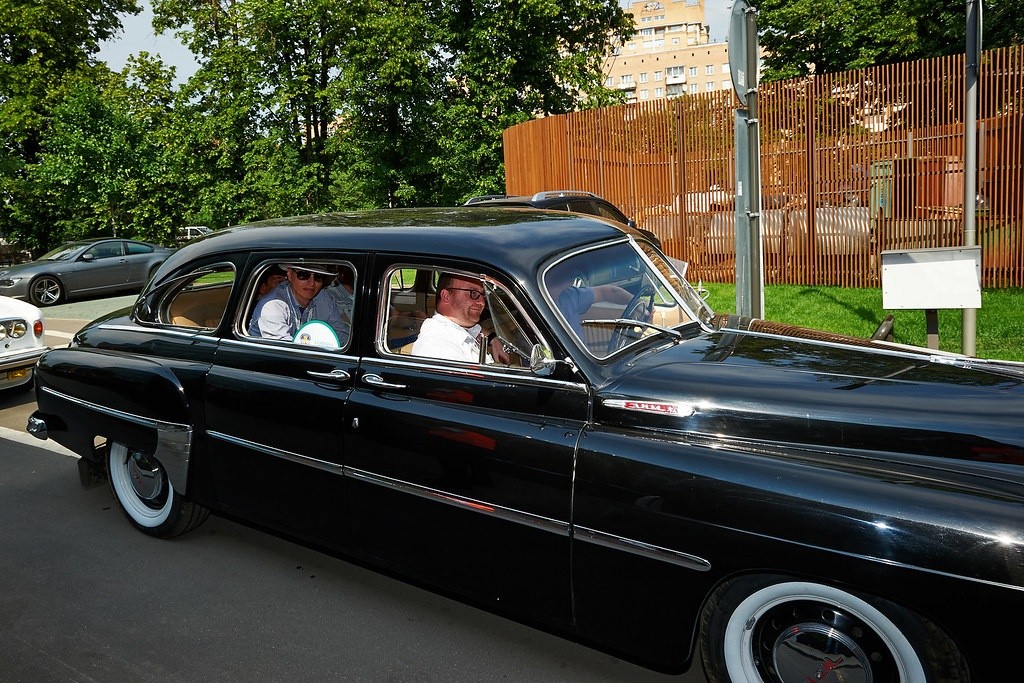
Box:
[{"left": 278, "top": 263, "right": 339, "bottom": 277}]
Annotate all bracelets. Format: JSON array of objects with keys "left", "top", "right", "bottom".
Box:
[{"left": 485, "top": 331, "right": 497, "bottom": 345}]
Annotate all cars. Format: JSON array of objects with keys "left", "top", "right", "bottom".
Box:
[
  {"left": 0, "top": 295, "right": 46, "bottom": 395},
  {"left": 26, "top": 205, "right": 1024, "bottom": 683},
  {"left": 176, "top": 226, "right": 214, "bottom": 244},
  {"left": 0, "top": 237, "right": 179, "bottom": 307}
]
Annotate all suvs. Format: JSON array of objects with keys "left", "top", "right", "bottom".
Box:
[{"left": 464, "top": 189, "right": 664, "bottom": 257}]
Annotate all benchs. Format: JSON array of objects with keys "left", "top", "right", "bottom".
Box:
[{"left": 169, "top": 282, "right": 233, "bottom": 328}]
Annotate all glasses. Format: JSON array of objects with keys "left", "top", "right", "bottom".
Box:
[
  {"left": 291, "top": 269, "right": 324, "bottom": 283},
  {"left": 447, "top": 287, "right": 487, "bottom": 300}
]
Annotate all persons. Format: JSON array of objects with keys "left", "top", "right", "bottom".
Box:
[
  {"left": 538, "top": 266, "right": 656, "bottom": 355},
  {"left": 411, "top": 272, "right": 513, "bottom": 373},
  {"left": 244, "top": 259, "right": 428, "bottom": 355}
]
